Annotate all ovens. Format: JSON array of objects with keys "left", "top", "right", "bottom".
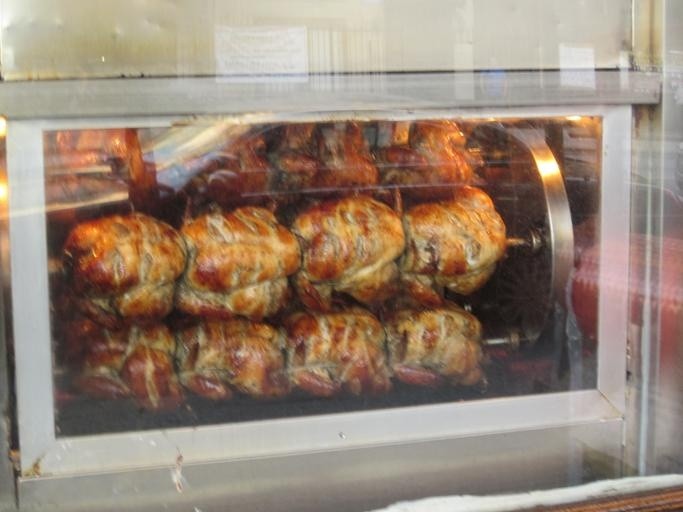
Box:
[{"left": 0, "top": 71, "right": 664, "bottom": 510}]
